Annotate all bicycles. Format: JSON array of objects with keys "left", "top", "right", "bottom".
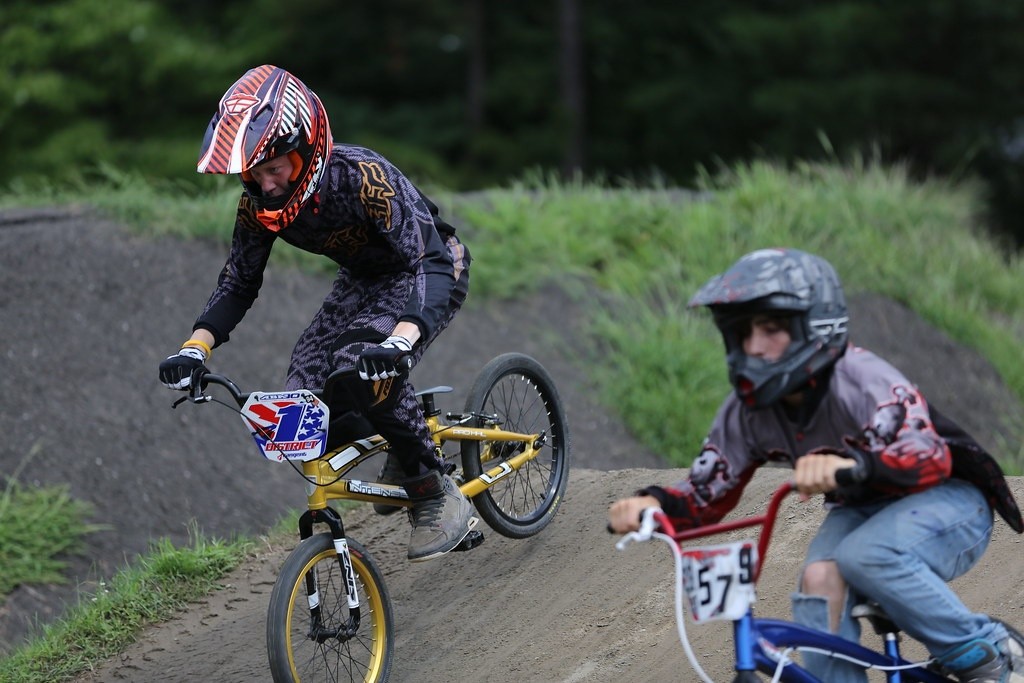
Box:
[
  {"left": 171, "top": 351, "right": 571, "bottom": 683},
  {"left": 607, "top": 466, "right": 1024, "bottom": 683}
]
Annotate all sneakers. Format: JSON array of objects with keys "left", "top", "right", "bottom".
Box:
[
  {"left": 373, "top": 444, "right": 407, "bottom": 514},
  {"left": 401, "top": 468, "right": 481, "bottom": 562}
]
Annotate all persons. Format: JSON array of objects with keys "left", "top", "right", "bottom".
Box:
[
  {"left": 606, "top": 246, "right": 1023, "bottom": 683},
  {"left": 157, "top": 63, "right": 484, "bottom": 562}
]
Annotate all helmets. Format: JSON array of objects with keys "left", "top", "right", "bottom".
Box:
[
  {"left": 686, "top": 246, "right": 849, "bottom": 411},
  {"left": 197, "top": 64, "right": 333, "bottom": 232}
]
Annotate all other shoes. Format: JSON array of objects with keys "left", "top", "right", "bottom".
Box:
[{"left": 937, "top": 614, "right": 1024, "bottom": 683}]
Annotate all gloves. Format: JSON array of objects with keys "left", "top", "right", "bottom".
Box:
[
  {"left": 356, "top": 335, "right": 412, "bottom": 381},
  {"left": 158, "top": 346, "right": 208, "bottom": 391}
]
